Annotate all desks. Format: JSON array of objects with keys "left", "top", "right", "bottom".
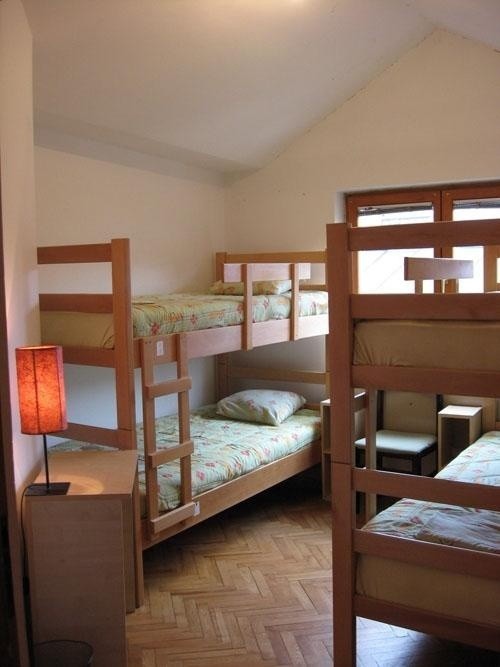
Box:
[{"left": 25, "top": 447, "right": 141, "bottom": 666}]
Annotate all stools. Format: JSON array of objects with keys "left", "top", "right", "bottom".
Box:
[{"left": 355, "top": 430, "right": 438, "bottom": 497}]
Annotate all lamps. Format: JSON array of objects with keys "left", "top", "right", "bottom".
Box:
[{"left": 14, "top": 341, "right": 73, "bottom": 497}]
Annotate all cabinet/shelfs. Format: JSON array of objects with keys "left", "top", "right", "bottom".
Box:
[
  {"left": 323, "top": 389, "right": 371, "bottom": 504},
  {"left": 439, "top": 407, "right": 482, "bottom": 470}
]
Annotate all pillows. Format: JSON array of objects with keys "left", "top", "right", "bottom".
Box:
[
  {"left": 214, "top": 389, "right": 307, "bottom": 425},
  {"left": 210, "top": 273, "right": 292, "bottom": 295}
]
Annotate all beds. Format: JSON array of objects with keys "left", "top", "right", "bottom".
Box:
[
  {"left": 328, "top": 224, "right": 500, "bottom": 394},
  {"left": 334, "top": 427, "right": 500, "bottom": 664},
  {"left": 42, "top": 370, "right": 328, "bottom": 610},
  {"left": 38, "top": 237, "right": 329, "bottom": 365}
]
